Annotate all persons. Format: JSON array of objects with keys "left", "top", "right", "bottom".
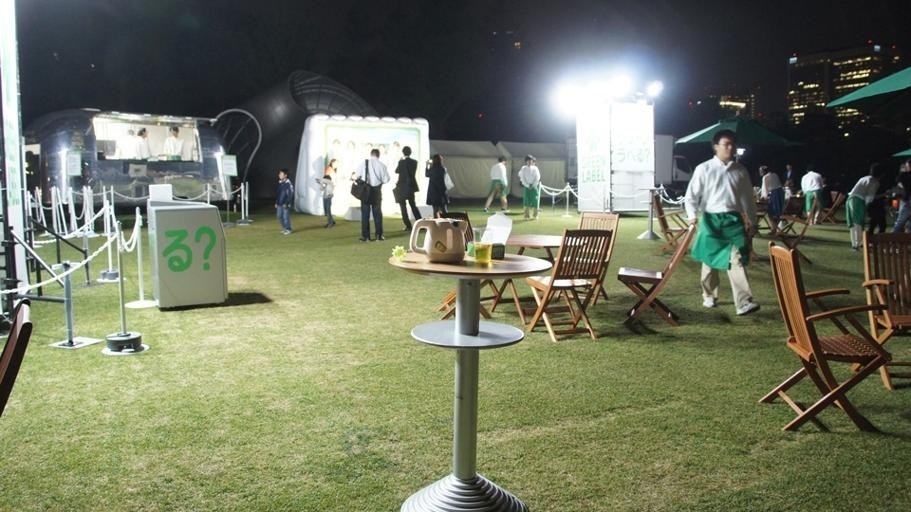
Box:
[
  {"left": 136, "top": 127, "right": 149, "bottom": 160},
  {"left": 316, "top": 175, "right": 336, "bottom": 228},
  {"left": 519, "top": 154, "right": 541, "bottom": 220},
  {"left": 324, "top": 159, "right": 338, "bottom": 178},
  {"left": 274, "top": 168, "right": 294, "bottom": 235},
  {"left": 683, "top": 129, "right": 761, "bottom": 316},
  {"left": 759, "top": 161, "right": 911, "bottom": 252},
  {"left": 349, "top": 149, "right": 390, "bottom": 242},
  {"left": 532, "top": 157, "right": 543, "bottom": 211},
  {"left": 164, "top": 126, "right": 183, "bottom": 162},
  {"left": 426, "top": 155, "right": 451, "bottom": 219},
  {"left": 392, "top": 147, "right": 421, "bottom": 231},
  {"left": 484, "top": 156, "right": 508, "bottom": 213}
]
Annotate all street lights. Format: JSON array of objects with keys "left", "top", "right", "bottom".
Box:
[{"left": 544, "top": 60, "right": 663, "bottom": 215}]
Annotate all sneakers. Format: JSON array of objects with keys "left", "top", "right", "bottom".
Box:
[
  {"left": 767, "top": 220, "right": 876, "bottom": 252},
  {"left": 736, "top": 302, "right": 760, "bottom": 316},
  {"left": 702, "top": 295, "right": 715, "bottom": 308},
  {"left": 280, "top": 206, "right": 539, "bottom": 242}
]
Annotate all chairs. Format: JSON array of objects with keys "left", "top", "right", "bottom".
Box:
[
  {"left": 0, "top": 296, "right": 35, "bottom": 416},
  {"left": 526, "top": 227, "right": 616, "bottom": 344},
  {"left": 759, "top": 239, "right": 893, "bottom": 434},
  {"left": 850, "top": 218, "right": 910, "bottom": 388},
  {"left": 617, "top": 224, "right": 695, "bottom": 331},
  {"left": 573, "top": 209, "right": 622, "bottom": 310},
  {"left": 435, "top": 210, "right": 495, "bottom": 321},
  {"left": 649, "top": 163, "right": 844, "bottom": 255}
]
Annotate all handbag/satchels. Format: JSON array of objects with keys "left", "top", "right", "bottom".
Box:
[
  {"left": 444, "top": 172, "right": 455, "bottom": 190},
  {"left": 352, "top": 178, "right": 376, "bottom": 204},
  {"left": 393, "top": 184, "right": 411, "bottom": 203}
]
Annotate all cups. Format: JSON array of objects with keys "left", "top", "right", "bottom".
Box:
[{"left": 472, "top": 226, "right": 495, "bottom": 263}]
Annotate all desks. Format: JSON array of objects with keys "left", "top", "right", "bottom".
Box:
[
  {"left": 470, "top": 233, "right": 588, "bottom": 322},
  {"left": 389, "top": 248, "right": 552, "bottom": 512}
]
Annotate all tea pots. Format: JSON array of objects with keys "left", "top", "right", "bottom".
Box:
[{"left": 409, "top": 217, "right": 469, "bottom": 265}]
